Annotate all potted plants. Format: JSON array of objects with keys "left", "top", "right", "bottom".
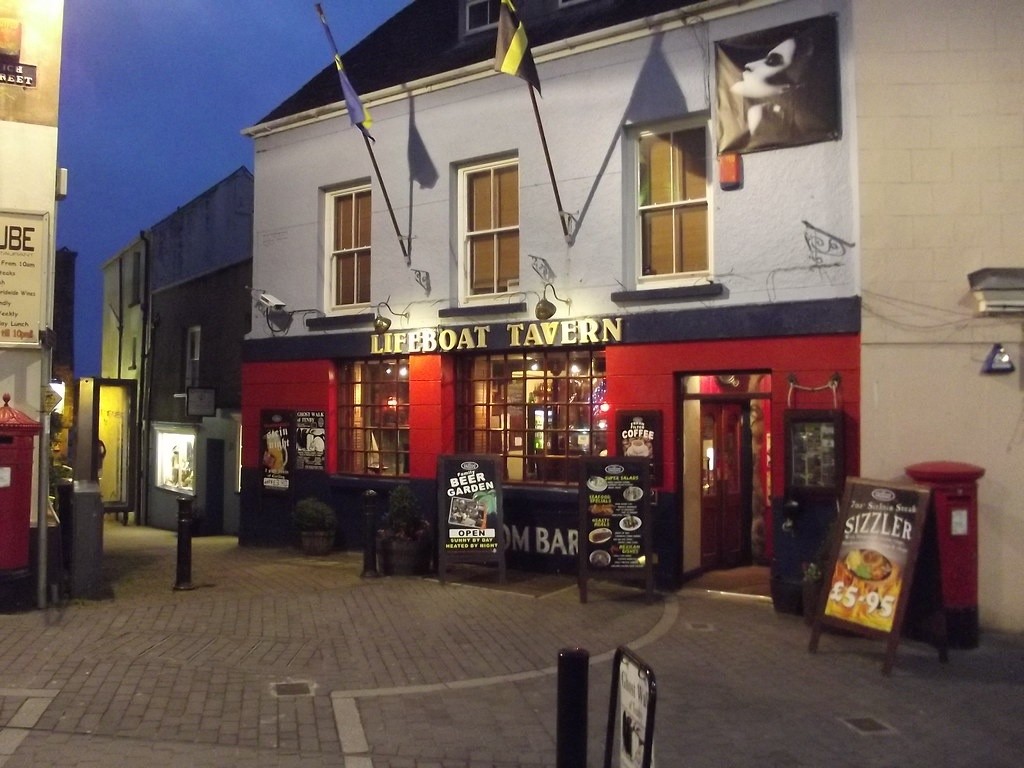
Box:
[
  {"left": 289, "top": 497, "right": 338, "bottom": 556},
  {"left": 375, "top": 483, "right": 430, "bottom": 573}
]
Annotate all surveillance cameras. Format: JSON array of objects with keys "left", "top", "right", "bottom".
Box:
[{"left": 259, "top": 294, "right": 286, "bottom": 311}]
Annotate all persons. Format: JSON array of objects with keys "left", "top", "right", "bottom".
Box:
[{"left": 723, "top": 32, "right": 838, "bottom": 153}]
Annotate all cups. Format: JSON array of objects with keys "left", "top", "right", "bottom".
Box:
[{"left": 632, "top": 440, "right": 644, "bottom": 453}]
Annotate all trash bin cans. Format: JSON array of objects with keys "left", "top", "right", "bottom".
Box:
[{"left": 767, "top": 493, "right": 839, "bottom": 616}]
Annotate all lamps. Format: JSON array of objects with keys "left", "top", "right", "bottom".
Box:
[
  {"left": 535, "top": 283, "right": 571, "bottom": 319},
  {"left": 374, "top": 302, "right": 409, "bottom": 335}
]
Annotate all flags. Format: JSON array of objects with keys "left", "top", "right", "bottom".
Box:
[
  {"left": 489, "top": 0, "right": 548, "bottom": 97},
  {"left": 317, "top": 12, "right": 384, "bottom": 144}
]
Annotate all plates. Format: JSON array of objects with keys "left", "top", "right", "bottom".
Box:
[
  {"left": 845, "top": 549, "right": 892, "bottom": 580},
  {"left": 627, "top": 445, "right": 649, "bottom": 457},
  {"left": 619, "top": 516, "right": 641, "bottom": 530},
  {"left": 589, "top": 528, "right": 612, "bottom": 543},
  {"left": 623, "top": 487, "right": 643, "bottom": 501},
  {"left": 590, "top": 549, "right": 611, "bottom": 564},
  {"left": 587, "top": 477, "right": 607, "bottom": 491}
]
